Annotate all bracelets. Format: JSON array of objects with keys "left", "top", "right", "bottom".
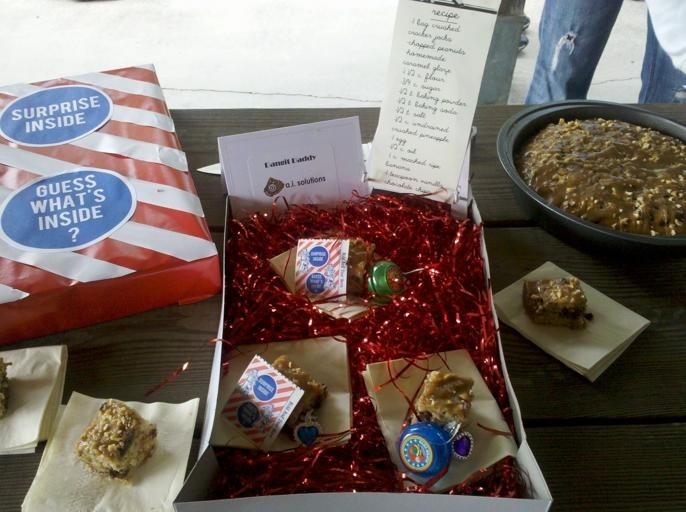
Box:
[{"left": 495, "top": 99, "right": 686, "bottom": 250}]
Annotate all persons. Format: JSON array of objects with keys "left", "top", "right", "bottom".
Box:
[{"left": 524, "top": 0, "right": 686, "bottom": 115}]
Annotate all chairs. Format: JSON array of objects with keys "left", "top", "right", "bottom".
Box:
[
  {"left": 519, "top": 35, "right": 529, "bottom": 51},
  {"left": 522, "top": 14, "right": 531, "bottom": 30}
]
